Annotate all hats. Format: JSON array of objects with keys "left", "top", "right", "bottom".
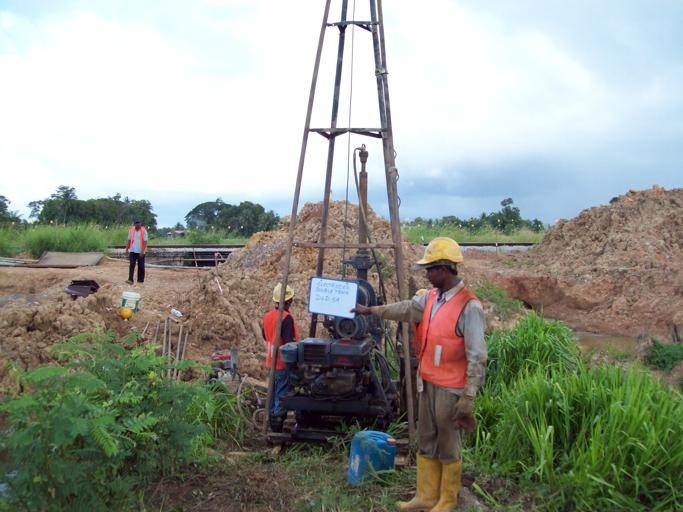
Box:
[{"left": 133, "top": 217, "right": 141, "bottom": 224}]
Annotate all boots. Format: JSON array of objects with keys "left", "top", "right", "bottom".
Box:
[
  {"left": 391, "top": 448, "right": 443, "bottom": 511},
  {"left": 427, "top": 455, "right": 463, "bottom": 512}
]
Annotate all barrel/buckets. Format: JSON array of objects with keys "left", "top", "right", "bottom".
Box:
[
  {"left": 347, "top": 430, "right": 399, "bottom": 488},
  {"left": 120, "top": 291, "right": 141, "bottom": 313}
]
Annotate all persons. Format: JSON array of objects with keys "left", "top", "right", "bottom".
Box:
[
  {"left": 261, "top": 282, "right": 299, "bottom": 443},
  {"left": 124, "top": 218, "right": 148, "bottom": 285},
  {"left": 348, "top": 236, "right": 489, "bottom": 512}
]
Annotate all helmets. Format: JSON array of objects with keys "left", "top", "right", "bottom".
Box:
[
  {"left": 272, "top": 282, "right": 295, "bottom": 303},
  {"left": 414, "top": 235, "right": 465, "bottom": 267}
]
